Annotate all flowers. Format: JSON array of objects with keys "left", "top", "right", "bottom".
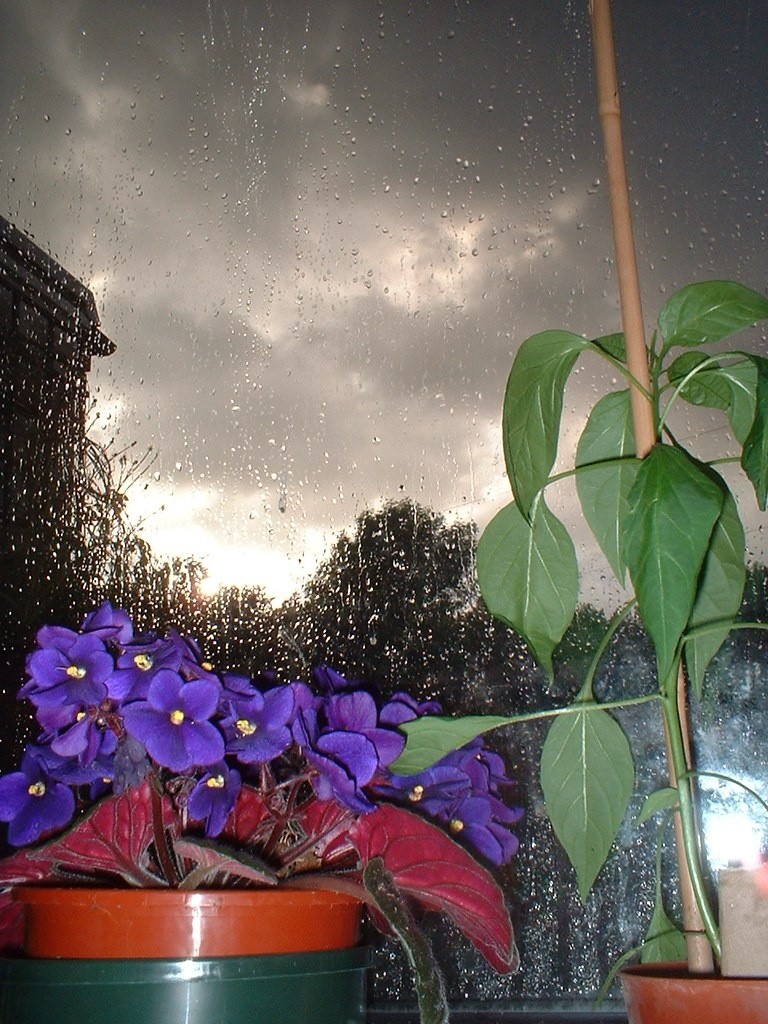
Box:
[{"left": 0, "top": 599, "right": 526, "bottom": 1023}]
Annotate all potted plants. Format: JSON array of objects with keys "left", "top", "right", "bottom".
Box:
[
  {"left": 401, "top": 281, "right": 768, "bottom": 1024},
  {"left": 12, "top": 886, "right": 360, "bottom": 958}
]
